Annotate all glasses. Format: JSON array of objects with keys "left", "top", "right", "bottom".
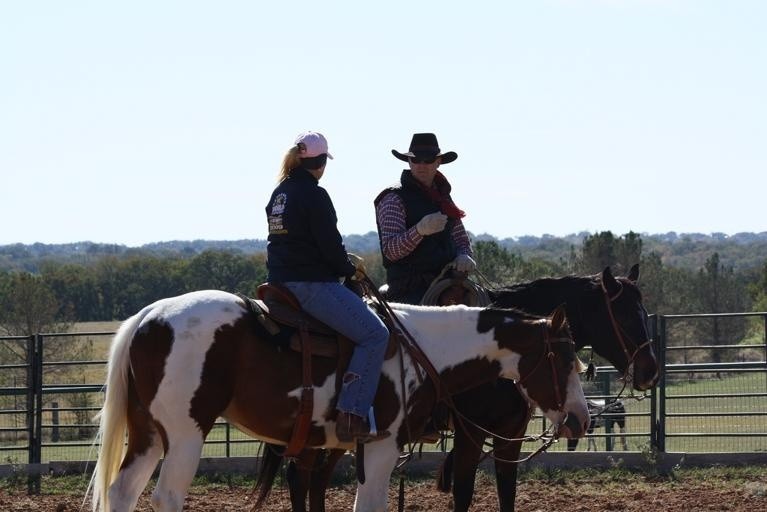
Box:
[{"left": 411, "top": 157, "right": 435, "bottom": 163}]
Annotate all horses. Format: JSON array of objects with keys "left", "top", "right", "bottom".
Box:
[
  {"left": 242, "top": 263, "right": 660, "bottom": 512},
  {"left": 567, "top": 395, "right": 628, "bottom": 452},
  {"left": 81, "top": 288, "right": 591, "bottom": 512}
]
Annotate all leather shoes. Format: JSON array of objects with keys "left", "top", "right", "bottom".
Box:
[{"left": 334, "top": 414, "right": 390, "bottom": 442}]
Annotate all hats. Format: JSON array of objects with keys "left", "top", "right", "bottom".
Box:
[
  {"left": 294, "top": 131, "right": 333, "bottom": 159},
  {"left": 392, "top": 134, "right": 456, "bottom": 164}
]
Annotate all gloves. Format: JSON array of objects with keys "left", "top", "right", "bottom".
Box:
[
  {"left": 453, "top": 254, "right": 476, "bottom": 272},
  {"left": 415, "top": 211, "right": 447, "bottom": 235}
]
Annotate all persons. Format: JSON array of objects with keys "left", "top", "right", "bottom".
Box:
[
  {"left": 371, "top": 132, "right": 477, "bottom": 305},
  {"left": 264, "top": 130, "right": 390, "bottom": 445}
]
